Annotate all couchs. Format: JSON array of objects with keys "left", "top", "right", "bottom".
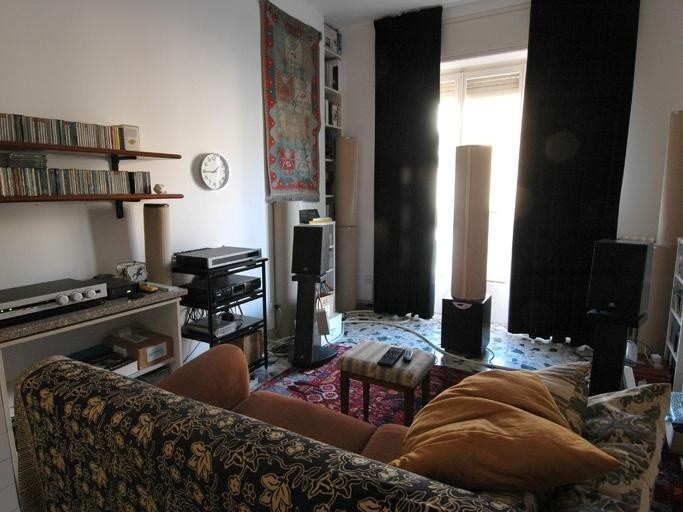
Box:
[{"left": 11, "top": 341, "right": 682, "bottom": 511}]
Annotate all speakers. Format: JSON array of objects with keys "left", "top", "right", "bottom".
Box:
[
  {"left": 585, "top": 238, "right": 654, "bottom": 318},
  {"left": 439, "top": 294, "right": 492, "bottom": 359},
  {"left": 449, "top": 144, "right": 491, "bottom": 302},
  {"left": 290, "top": 224, "right": 328, "bottom": 277}
]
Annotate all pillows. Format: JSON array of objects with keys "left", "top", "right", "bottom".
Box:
[
  {"left": 557, "top": 385, "right": 673, "bottom": 512},
  {"left": 482, "top": 360, "right": 591, "bottom": 512},
  {"left": 386, "top": 369, "right": 624, "bottom": 481}
]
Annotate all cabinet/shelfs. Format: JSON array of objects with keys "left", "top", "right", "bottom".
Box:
[
  {"left": 0, "top": 116, "right": 186, "bottom": 212},
  {"left": 319, "top": 11, "right": 346, "bottom": 217},
  {"left": 661, "top": 237, "right": 682, "bottom": 392}
]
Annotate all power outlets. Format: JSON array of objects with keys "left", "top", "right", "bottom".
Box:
[{"left": 270, "top": 302, "right": 280, "bottom": 311}]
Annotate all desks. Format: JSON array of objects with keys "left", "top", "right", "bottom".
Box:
[{"left": 0, "top": 276, "right": 188, "bottom": 481}]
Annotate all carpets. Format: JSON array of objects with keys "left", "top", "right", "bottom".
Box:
[{"left": 250, "top": 339, "right": 682, "bottom": 511}]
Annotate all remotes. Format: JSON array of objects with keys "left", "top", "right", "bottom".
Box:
[
  {"left": 377, "top": 347, "right": 404, "bottom": 368},
  {"left": 403, "top": 348, "right": 413, "bottom": 363}
]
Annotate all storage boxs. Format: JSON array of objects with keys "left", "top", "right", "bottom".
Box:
[
  {"left": 102, "top": 325, "right": 174, "bottom": 370},
  {"left": 241, "top": 332, "right": 261, "bottom": 361}
]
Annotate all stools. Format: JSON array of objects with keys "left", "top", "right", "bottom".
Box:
[{"left": 337, "top": 340, "right": 435, "bottom": 426}]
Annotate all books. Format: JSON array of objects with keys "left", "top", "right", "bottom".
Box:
[
  {"left": 67, "top": 343, "right": 139, "bottom": 376},
  {"left": 324, "top": 99, "right": 339, "bottom": 126},
  {"left": 0, "top": 153, "right": 152, "bottom": 196},
  {"left": 0, "top": 112, "right": 141, "bottom": 151}
]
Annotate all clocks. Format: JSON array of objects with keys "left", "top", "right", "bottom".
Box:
[{"left": 199, "top": 154, "right": 229, "bottom": 190}]
